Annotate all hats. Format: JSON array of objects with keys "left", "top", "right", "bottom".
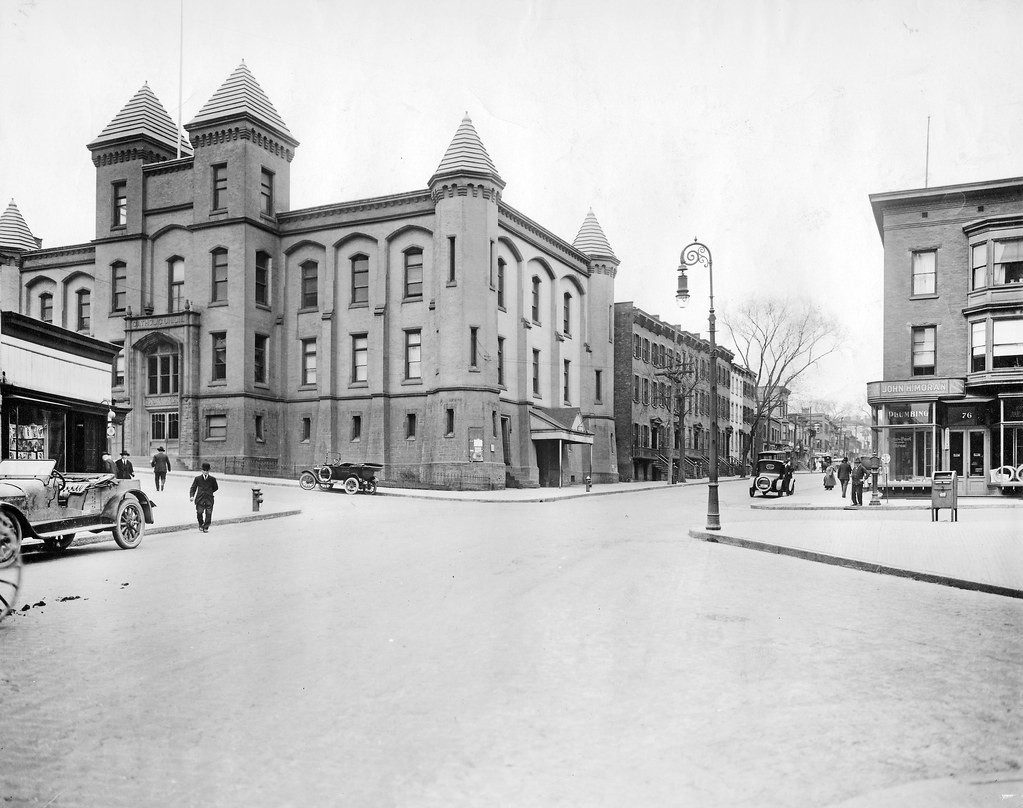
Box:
[
  {"left": 854, "top": 458, "right": 862, "bottom": 464},
  {"left": 156, "top": 446, "right": 165, "bottom": 453},
  {"left": 120, "top": 450, "right": 131, "bottom": 457},
  {"left": 843, "top": 456, "right": 848, "bottom": 461},
  {"left": 199, "top": 461, "right": 211, "bottom": 473}
]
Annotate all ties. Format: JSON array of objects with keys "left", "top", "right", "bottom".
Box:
[
  {"left": 205, "top": 475, "right": 207, "bottom": 479},
  {"left": 124, "top": 460, "right": 125, "bottom": 465}
]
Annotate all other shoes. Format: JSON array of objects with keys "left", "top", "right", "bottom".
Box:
[
  {"left": 203, "top": 525, "right": 209, "bottom": 533},
  {"left": 198, "top": 524, "right": 203, "bottom": 531},
  {"left": 857, "top": 503, "right": 862, "bottom": 507},
  {"left": 852, "top": 503, "right": 856, "bottom": 507}
]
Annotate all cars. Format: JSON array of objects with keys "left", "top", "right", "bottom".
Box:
[
  {"left": 750, "top": 451, "right": 795, "bottom": 497},
  {"left": 299, "top": 452, "right": 384, "bottom": 495},
  {"left": 0, "top": 459, "right": 156, "bottom": 568}
]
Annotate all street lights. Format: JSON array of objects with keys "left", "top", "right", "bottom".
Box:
[
  {"left": 676, "top": 236, "right": 719, "bottom": 531},
  {"left": 97, "top": 400, "right": 116, "bottom": 472}
]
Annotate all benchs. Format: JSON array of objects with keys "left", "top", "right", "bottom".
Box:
[{"left": 56, "top": 473, "right": 117, "bottom": 504}]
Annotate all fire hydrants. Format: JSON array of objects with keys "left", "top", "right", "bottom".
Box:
[
  {"left": 585, "top": 476, "right": 591, "bottom": 492},
  {"left": 252, "top": 487, "right": 262, "bottom": 513}
]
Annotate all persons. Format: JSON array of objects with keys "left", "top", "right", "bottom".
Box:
[
  {"left": 190, "top": 463, "right": 218, "bottom": 533},
  {"left": 151, "top": 446, "right": 171, "bottom": 491},
  {"left": 825, "top": 460, "right": 836, "bottom": 491},
  {"left": 102, "top": 452, "right": 119, "bottom": 479},
  {"left": 851, "top": 458, "right": 870, "bottom": 506},
  {"left": 837, "top": 457, "right": 852, "bottom": 498},
  {"left": 115, "top": 451, "right": 135, "bottom": 479},
  {"left": 10, "top": 426, "right": 43, "bottom": 459}
]
